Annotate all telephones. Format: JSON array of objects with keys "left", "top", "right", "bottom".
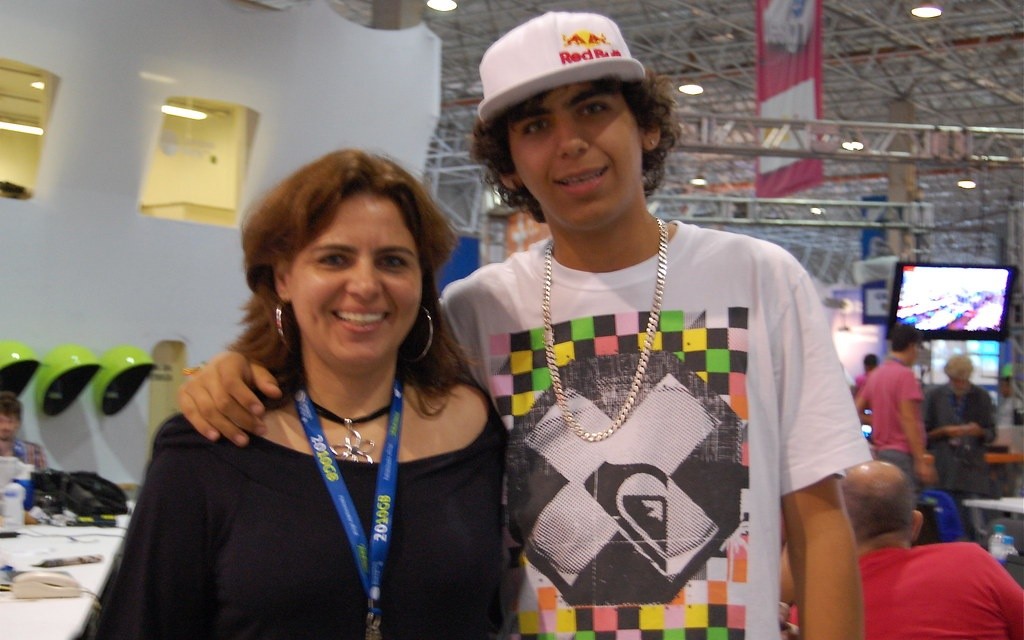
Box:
[{"left": 11, "top": 571, "right": 83, "bottom": 597}]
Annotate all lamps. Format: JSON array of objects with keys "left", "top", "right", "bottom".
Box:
[
  {"left": 911, "top": 0, "right": 941, "bottom": 18},
  {"left": 678, "top": 77, "right": 704, "bottom": 94}
]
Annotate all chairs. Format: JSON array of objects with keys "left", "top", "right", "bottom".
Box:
[{"left": 922, "top": 488, "right": 1023, "bottom": 580}]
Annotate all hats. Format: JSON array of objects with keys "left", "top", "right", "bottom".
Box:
[{"left": 477, "top": 10, "right": 647, "bottom": 124}]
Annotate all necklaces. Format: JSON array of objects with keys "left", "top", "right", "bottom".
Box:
[
  {"left": 540, "top": 215, "right": 667, "bottom": 442},
  {"left": 309, "top": 397, "right": 392, "bottom": 465}
]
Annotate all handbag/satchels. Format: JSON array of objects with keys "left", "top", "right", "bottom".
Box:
[{"left": 32, "top": 469, "right": 127, "bottom": 517}]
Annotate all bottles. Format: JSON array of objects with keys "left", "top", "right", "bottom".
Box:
[
  {"left": 986, "top": 524, "right": 1020, "bottom": 566},
  {"left": 2, "top": 478, "right": 26, "bottom": 525}
]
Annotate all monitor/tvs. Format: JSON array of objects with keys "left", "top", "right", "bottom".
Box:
[{"left": 886, "top": 262, "right": 1018, "bottom": 341}]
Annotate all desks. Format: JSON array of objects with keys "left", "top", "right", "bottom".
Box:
[
  {"left": 963, "top": 500, "right": 1024, "bottom": 539},
  {"left": 922, "top": 450, "right": 1024, "bottom": 524},
  {"left": 0, "top": 498, "right": 134, "bottom": 639}
]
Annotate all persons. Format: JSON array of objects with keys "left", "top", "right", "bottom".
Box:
[
  {"left": 779, "top": 324, "right": 1024, "bottom": 640},
  {"left": 0, "top": 393, "right": 50, "bottom": 525},
  {"left": 67, "top": 151, "right": 519, "bottom": 640},
  {"left": 177, "top": 12, "right": 879, "bottom": 640}
]
